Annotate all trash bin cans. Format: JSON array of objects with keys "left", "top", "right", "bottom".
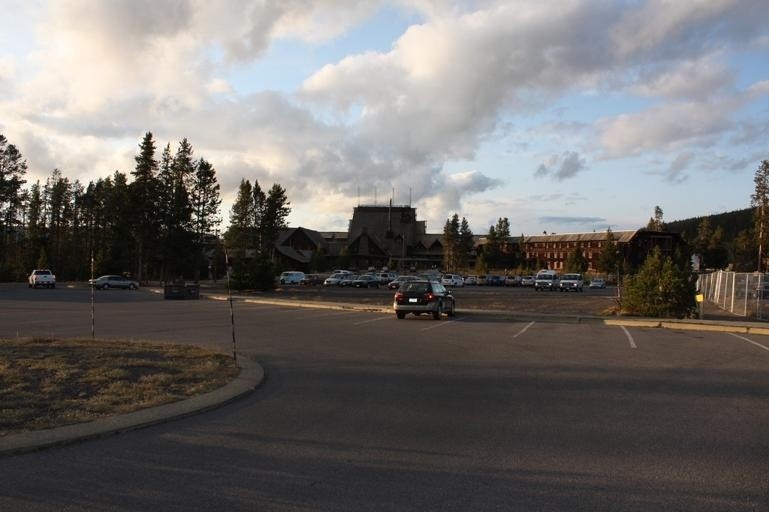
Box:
[{"left": 164, "top": 285, "right": 200, "bottom": 301}]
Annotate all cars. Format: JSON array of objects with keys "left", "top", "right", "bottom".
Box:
[
  {"left": 307, "top": 269, "right": 534, "bottom": 287},
  {"left": 393, "top": 279, "right": 455, "bottom": 319},
  {"left": 589, "top": 277, "right": 606, "bottom": 289},
  {"left": 89, "top": 275, "right": 140, "bottom": 290},
  {"left": 560, "top": 273, "right": 584, "bottom": 293},
  {"left": 28, "top": 269, "right": 56, "bottom": 289}
]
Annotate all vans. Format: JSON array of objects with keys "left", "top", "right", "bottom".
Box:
[
  {"left": 534, "top": 269, "right": 558, "bottom": 291},
  {"left": 280, "top": 271, "right": 306, "bottom": 285}
]
